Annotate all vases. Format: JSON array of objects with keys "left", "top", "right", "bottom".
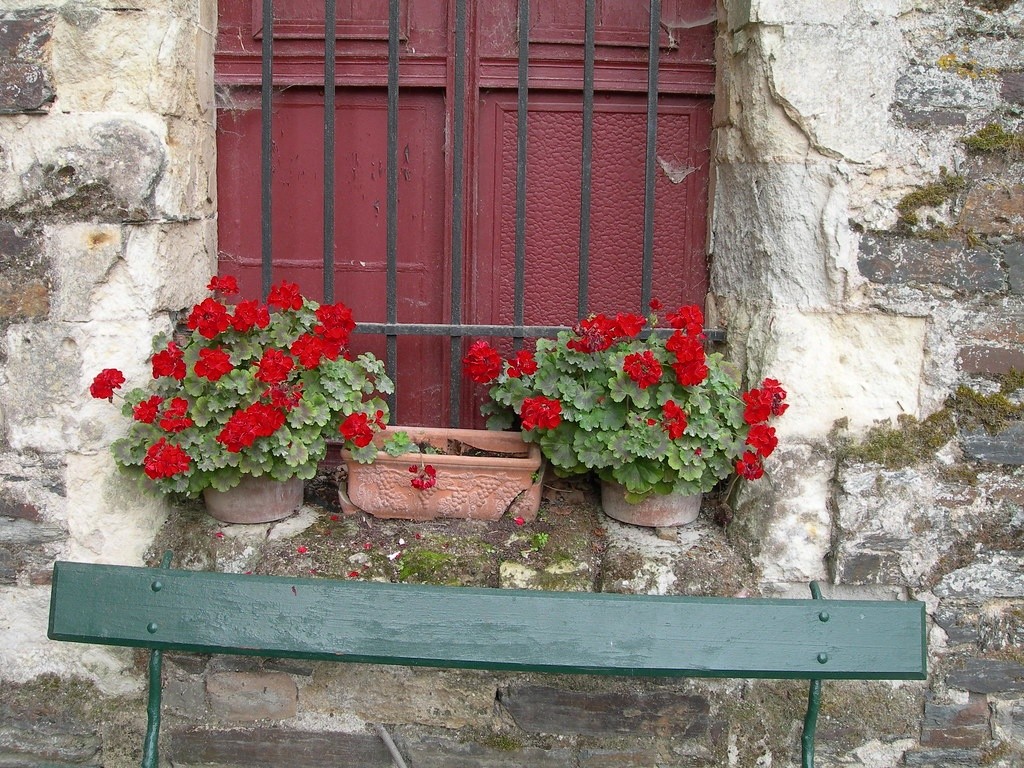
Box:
[
  {"left": 202, "top": 474, "right": 306, "bottom": 525},
  {"left": 600, "top": 474, "right": 703, "bottom": 528},
  {"left": 338, "top": 425, "right": 544, "bottom": 519}
]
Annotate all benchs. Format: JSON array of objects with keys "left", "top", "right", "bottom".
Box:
[{"left": 47, "top": 561, "right": 926, "bottom": 768}]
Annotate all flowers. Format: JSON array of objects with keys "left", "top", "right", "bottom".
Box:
[
  {"left": 88, "top": 274, "right": 396, "bottom": 501},
  {"left": 459, "top": 299, "right": 789, "bottom": 506},
  {"left": 380, "top": 432, "right": 437, "bottom": 490}
]
